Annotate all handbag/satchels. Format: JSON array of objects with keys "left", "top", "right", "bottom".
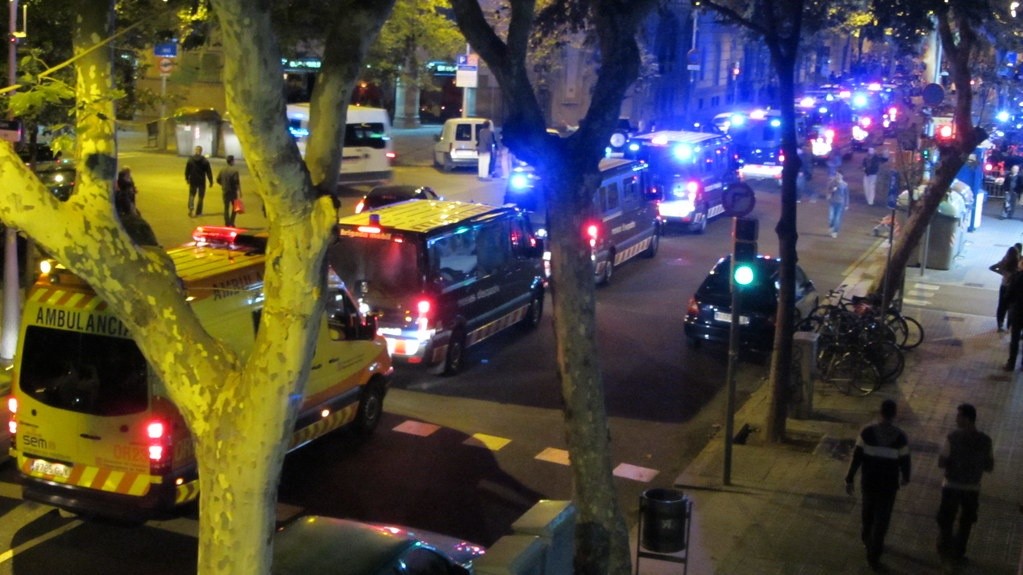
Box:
[{"left": 234, "top": 196, "right": 246, "bottom": 214}]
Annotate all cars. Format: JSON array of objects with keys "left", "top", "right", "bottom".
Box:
[
  {"left": 15, "top": 140, "right": 76, "bottom": 201},
  {"left": 952, "top": 72, "right": 1023, "bottom": 191},
  {"left": 683, "top": 252, "right": 819, "bottom": 347},
  {"left": 271, "top": 486, "right": 515, "bottom": 575}
]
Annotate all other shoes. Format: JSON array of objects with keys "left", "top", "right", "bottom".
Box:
[
  {"left": 1000, "top": 363, "right": 1012, "bottom": 371},
  {"left": 831, "top": 231, "right": 837, "bottom": 238},
  {"left": 479, "top": 177, "right": 492, "bottom": 182},
  {"left": 828, "top": 227, "right": 834, "bottom": 232},
  {"left": 997, "top": 328, "right": 1004, "bottom": 333},
  {"left": 187, "top": 207, "right": 194, "bottom": 216}
]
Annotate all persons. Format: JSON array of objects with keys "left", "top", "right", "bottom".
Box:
[
  {"left": 862, "top": 146, "right": 889, "bottom": 205},
  {"left": 844, "top": 399, "right": 912, "bottom": 566},
  {"left": 936, "top": 403, "right": 994, "bottom": 558},
  {"left": 827, "top": 170, "right": 850, "bottom": 238},
  {"left": 184, "top": 146, "right": 214, "bottom": 217},
  {"left": 1002, "top": 165, "right": 1023, "bottom": 219},
  {"left": 989, "top": 243, "right": 1023, "bottom": 372},
  {"left": 495, "top": 134, "right": 512, "bottom": 179},
  {"left": 477, "top": 121, "right": 494, "bottom": 182},
  {"left": 117, "top": 165, "right": 138, "bottom": 207},
  {"left": 216, "top": 155, "right": 242, "bottom": 227}
]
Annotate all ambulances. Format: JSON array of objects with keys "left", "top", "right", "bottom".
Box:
[{"left": 9, "top": 226, "right": 395, "bottom": 518}]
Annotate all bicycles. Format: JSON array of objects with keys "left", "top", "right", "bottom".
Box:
[{"left": 792, "top": 284, "right": 924, "bottom": 397}]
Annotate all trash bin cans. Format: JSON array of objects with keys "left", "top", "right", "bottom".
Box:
[
  {"left": 925, "top": 191, "right": 967, "bottom": 270},
  {"left": 223, "top": 112, "right": 244, "bottom": 159},
  {"left": 894, "top": 186, "right": 929, "bottom": 268},
  {"left": 948, "top": 178, "right": 974, "bottom": 257},
  {"left": 640, "top": 488, "right": 690, "bottom": 553},
  {"left": 173, "top": 107, "right": 223, "bottom": 158}
]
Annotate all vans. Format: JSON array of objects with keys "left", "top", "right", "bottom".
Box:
[
  {"left": 504, "top": 161, "right": 667, "bottom": 288},
  {"left": 434, "top": 116, "right": 495, "bottom": 173},
  {"left": 329, "top": 195, "right": 545, "bottom": 378},
  {"left": 627, "top": 131, "right": 742, "bottom": 235},
  {"left": 713, "top": 74, "right": 918, "bottom": 188},
  {"left": 284, "top": 102, "right": 393, "bottom": 190}
]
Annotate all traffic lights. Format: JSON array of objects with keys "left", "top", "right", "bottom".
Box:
[{"left": 734, "top": 219, "right": 758, "bottom": 288}]
